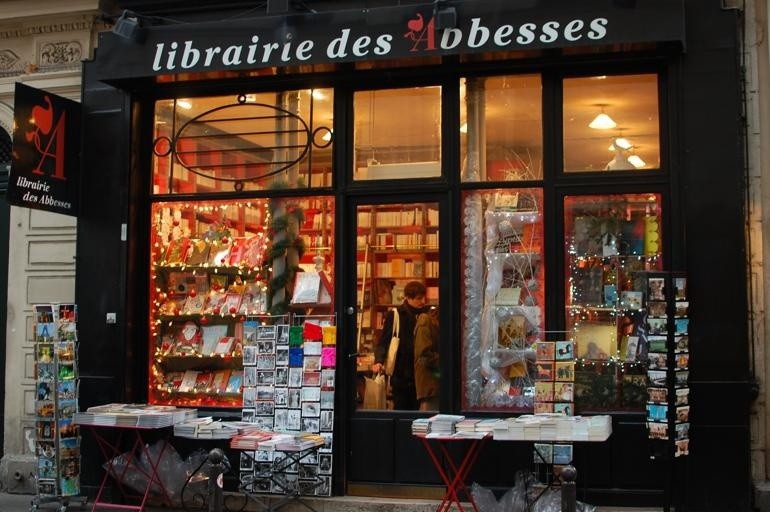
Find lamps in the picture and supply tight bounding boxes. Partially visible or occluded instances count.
[588,107,646,169]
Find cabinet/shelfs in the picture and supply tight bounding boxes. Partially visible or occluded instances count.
[156,122,440,406]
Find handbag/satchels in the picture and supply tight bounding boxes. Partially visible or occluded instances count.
[384,307,401,377]
[361,369,388,411]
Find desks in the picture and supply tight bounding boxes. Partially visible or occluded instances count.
[416,434,613,512]
[76,423,326,512]
